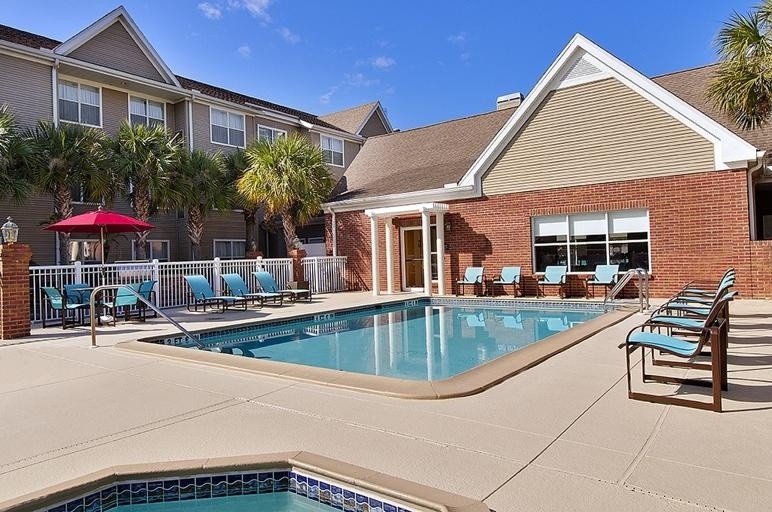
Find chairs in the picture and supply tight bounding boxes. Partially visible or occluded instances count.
[455,266,489,298]
[618,265,741,414]
[252,271,313,304]
[38,278,160,331]
[182,274,245,314]
[456,306,586,334]
[535,265,573,299]
[492,265,526,299]
[584,263,619,304]
[218,273,284,309]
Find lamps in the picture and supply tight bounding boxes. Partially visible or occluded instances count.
[1,215,20,244]
[292,235,302,250]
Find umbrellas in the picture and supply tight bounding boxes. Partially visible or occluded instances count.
[40,206,156,316]
